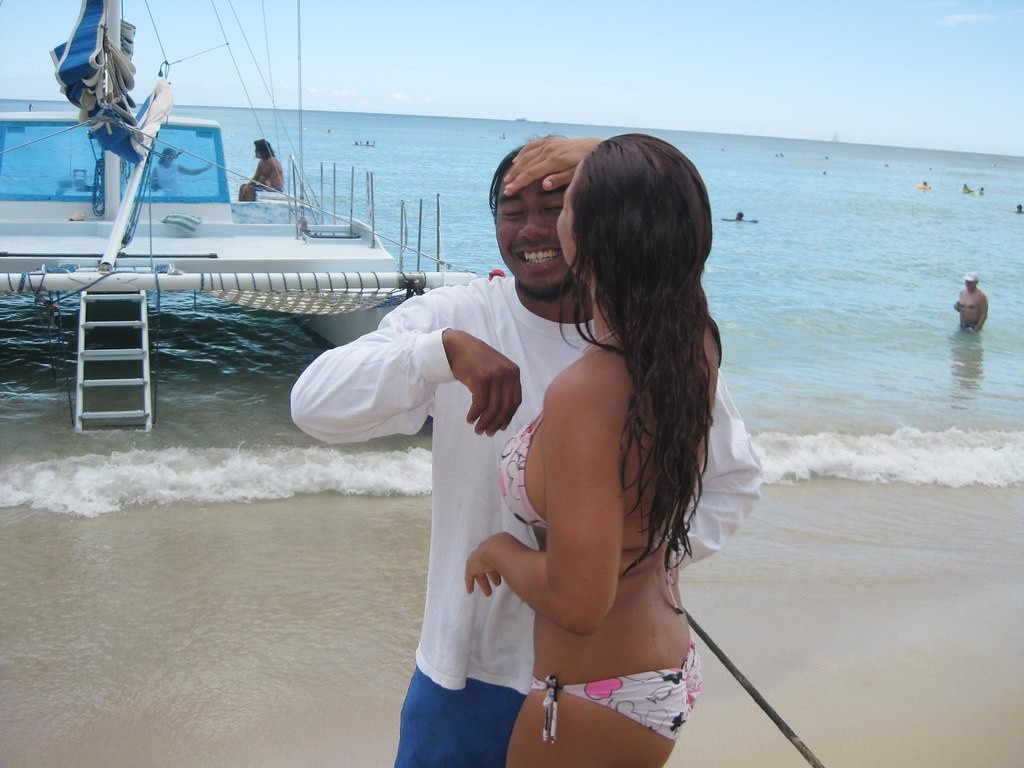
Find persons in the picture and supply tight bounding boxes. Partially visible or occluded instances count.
[960,184,972,193]
[1014,204,1024,213]
[238,137,284,203]
[952,273,989,333]
[922,181,928,187]
[722,211,759,224]
[978,187,984,196]
[290,133,765,768]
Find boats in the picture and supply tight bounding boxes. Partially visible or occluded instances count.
[1,0,505,419]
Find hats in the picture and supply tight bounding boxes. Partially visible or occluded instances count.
[964,271,979,281]
